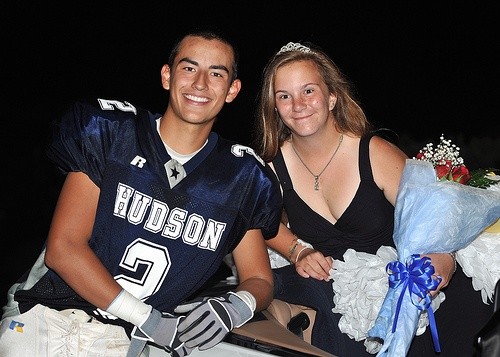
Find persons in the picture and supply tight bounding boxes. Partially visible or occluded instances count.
[0,26,284,357]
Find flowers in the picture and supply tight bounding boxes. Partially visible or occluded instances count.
[364,133,500,357]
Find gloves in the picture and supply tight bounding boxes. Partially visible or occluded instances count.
[173,292,254,351]
[127,309,195,357]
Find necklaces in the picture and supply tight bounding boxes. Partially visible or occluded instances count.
[449,252,458,274]
[289,135,345,192]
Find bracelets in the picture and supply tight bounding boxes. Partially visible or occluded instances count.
[250,40,500,357]
[287,237,312,264]
